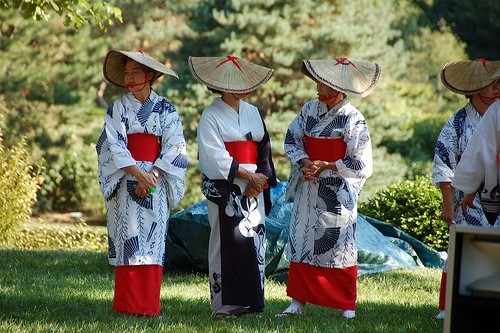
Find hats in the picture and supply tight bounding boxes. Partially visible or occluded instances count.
[301,56,381,99]
[188,54,275,94]
[442,58,500,97]
[102,49,179,88]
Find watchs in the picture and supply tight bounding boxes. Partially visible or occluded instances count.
[152,171,158,178]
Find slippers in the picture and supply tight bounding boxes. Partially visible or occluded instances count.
[275,311,306,319]
[341,316,357,322]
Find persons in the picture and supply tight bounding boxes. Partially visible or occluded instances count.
[432,61,500,318]
[189,56,278,317]
[284,57,380,318]
[451,100,500,214]
[97,49,187,317]
